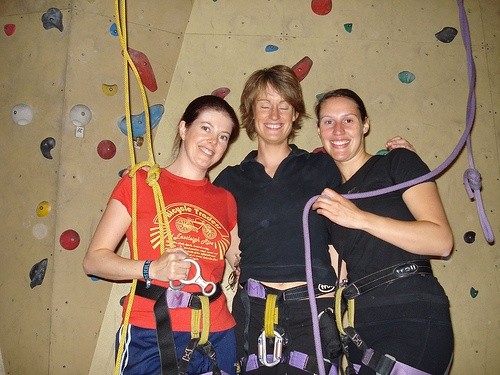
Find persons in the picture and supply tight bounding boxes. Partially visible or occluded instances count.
[82,95,243,375]
[313,86,456,375]
[119,63,414,375]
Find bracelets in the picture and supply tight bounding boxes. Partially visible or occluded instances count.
[142,259,155,289]
